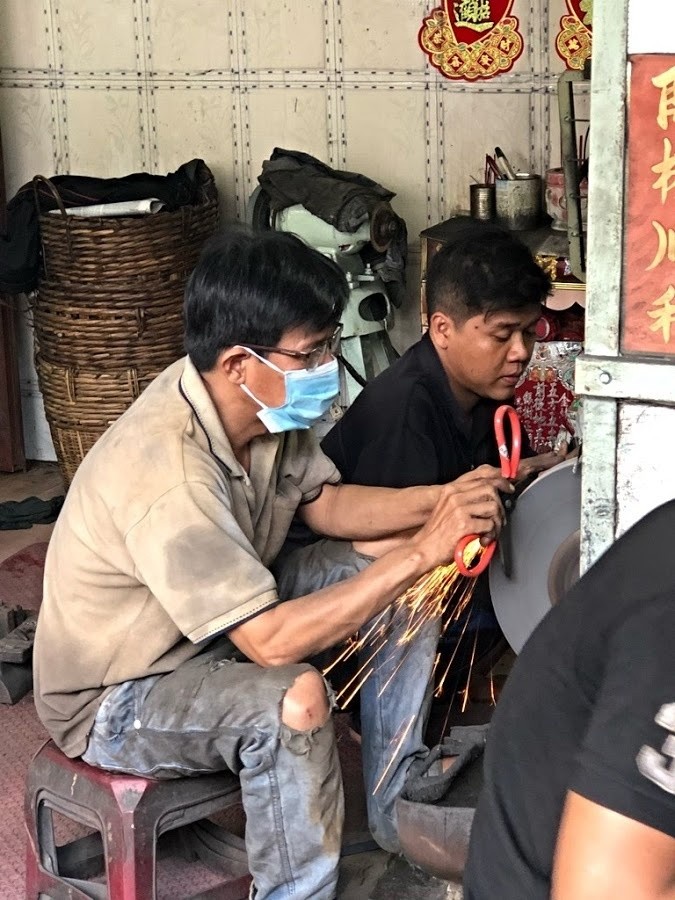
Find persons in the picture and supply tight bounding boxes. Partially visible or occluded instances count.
[30,225,517,900]
[320,217,582,736]
[462,496,675,899]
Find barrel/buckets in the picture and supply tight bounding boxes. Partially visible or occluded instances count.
[496,173,544,232]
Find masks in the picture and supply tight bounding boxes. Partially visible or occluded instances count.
[232,343,341,435]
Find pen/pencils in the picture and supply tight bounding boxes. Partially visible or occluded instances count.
[469,146,518,185]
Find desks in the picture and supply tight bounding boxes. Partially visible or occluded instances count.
[420,215,587,346]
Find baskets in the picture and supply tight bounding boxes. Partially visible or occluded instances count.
[23,170,226,499]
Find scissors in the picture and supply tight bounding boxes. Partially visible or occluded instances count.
[454,404,532,580]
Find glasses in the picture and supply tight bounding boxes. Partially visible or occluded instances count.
[230,322,345,374]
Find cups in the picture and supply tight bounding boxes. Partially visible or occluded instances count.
[543,165,587,233]
[469,184,494,221]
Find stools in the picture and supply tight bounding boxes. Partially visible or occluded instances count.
[24,737,252,900]
